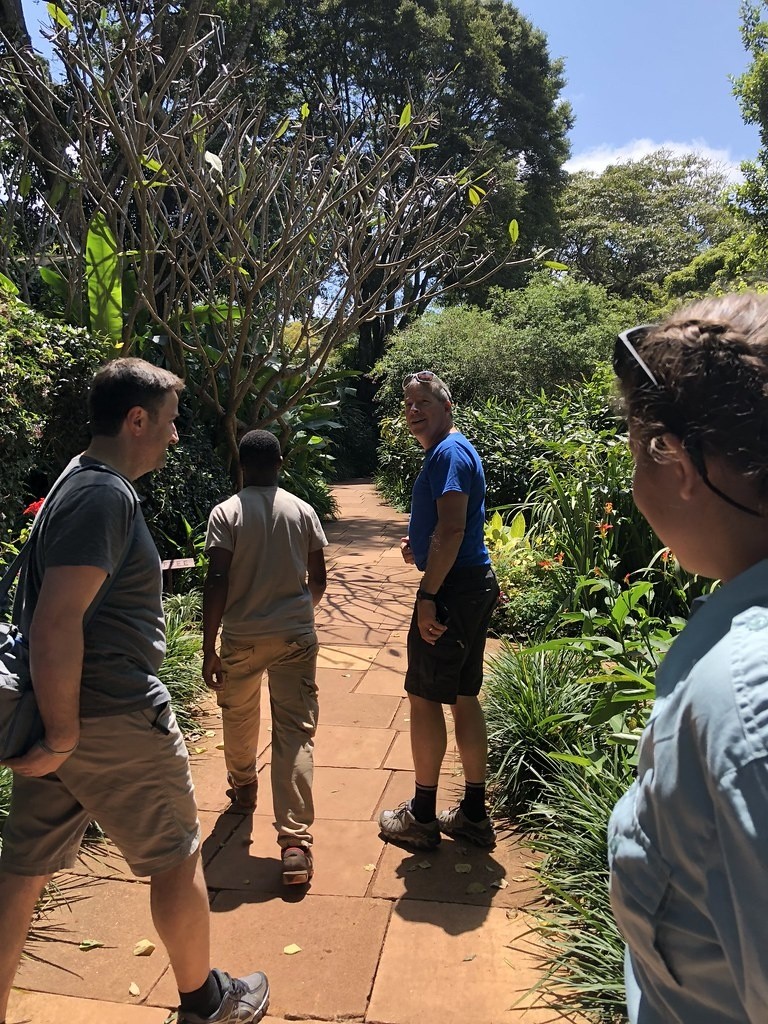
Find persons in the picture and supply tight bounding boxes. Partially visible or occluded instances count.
[604,298,768,1024]
[378,372,501,847]
[0,357,272,1024]
[201,431,330,883]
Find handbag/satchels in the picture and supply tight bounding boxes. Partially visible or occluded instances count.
[0,613,44,761]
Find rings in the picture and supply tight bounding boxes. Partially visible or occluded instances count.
[428,627,433,631]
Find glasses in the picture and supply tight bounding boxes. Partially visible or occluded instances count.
[612,324,660,389]
[402,370,452,403]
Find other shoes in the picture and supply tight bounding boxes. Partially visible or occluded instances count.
[234,790,257,813]
[280,846,314,884]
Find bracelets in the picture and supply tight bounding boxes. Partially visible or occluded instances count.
[37,738,80,755]
[415,589,435,601]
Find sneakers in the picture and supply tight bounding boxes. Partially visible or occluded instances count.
[175,967,270,1024]
[378,798,442,848]
[439,800,497,846]
